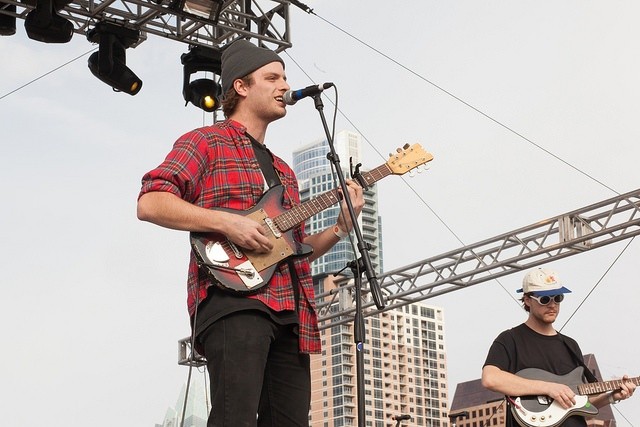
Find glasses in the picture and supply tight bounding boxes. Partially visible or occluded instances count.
[529,294,564,306]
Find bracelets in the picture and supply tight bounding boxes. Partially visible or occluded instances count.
[332,223,352,240]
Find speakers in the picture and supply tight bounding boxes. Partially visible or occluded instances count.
[449,354,616,427]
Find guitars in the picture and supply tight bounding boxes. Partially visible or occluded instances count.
[509,365,640,427]
[189,141,436,296]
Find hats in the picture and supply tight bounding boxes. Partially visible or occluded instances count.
[516,267,572,297]
[220,38,286,97]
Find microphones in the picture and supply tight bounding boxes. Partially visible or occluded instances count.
[197,261,255,277]
[283,82,333,104]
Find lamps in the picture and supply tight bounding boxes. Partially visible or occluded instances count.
[86,21,143,96]
[23,7,75,43]
[182,46,225,112]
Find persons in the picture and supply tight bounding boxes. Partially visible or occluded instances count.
[482,268,636,427]
[136,41,366,427]
